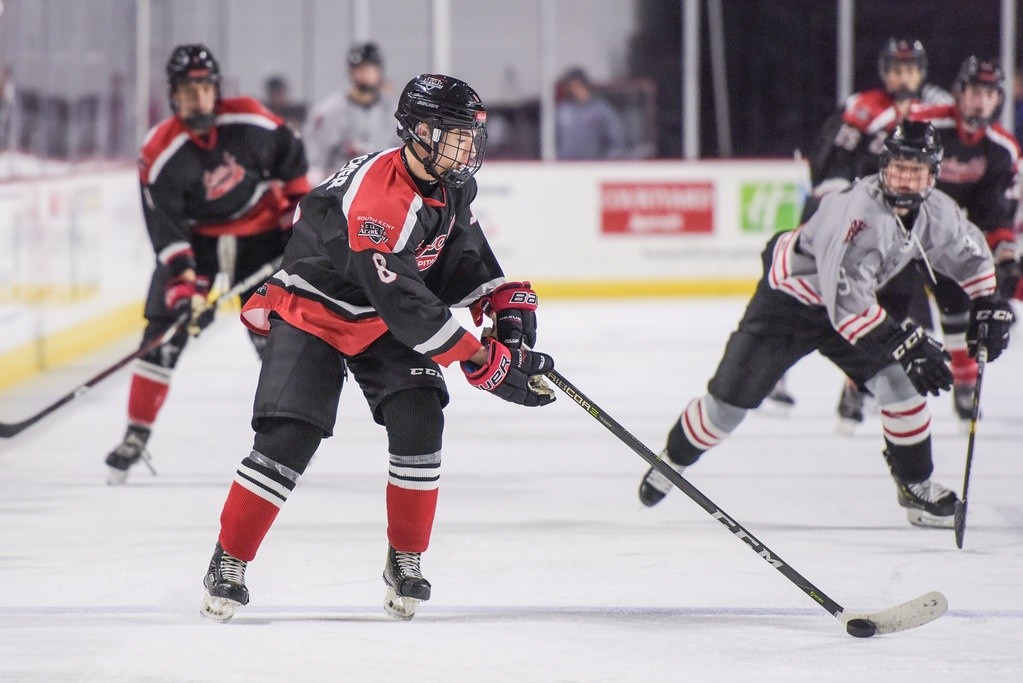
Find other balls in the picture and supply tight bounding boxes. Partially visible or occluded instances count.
[847,618,876,637]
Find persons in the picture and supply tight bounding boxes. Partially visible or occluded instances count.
[744,36,959,416]
[556,69,633,160]
[642,56,714,158]
[0,63,21,108]
[302,45,403,189]
[639,115,1013,528]
[104,45,313,487]
[198,70,559,624]
[836,50,1023,434]
[260,74,306,128]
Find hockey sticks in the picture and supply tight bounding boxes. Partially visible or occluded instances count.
[1,257,284,442]
[953,321,991,550]
[546,366,950,637]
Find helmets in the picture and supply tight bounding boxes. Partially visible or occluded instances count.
[951,52,1005,126]
[166,44,219,129]
[878,36,927,96]
[876,120,942,210]
[348,44,379,66]
[394,74,488,190]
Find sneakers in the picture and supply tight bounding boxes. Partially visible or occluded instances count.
[382,544,431,620]
[198,541,249,622]
[104,424,150,483]
[763,380,797,416]
[639,449,686,507]
[882,451,960,529]
[838,378,866,421]
[954,385,982,427]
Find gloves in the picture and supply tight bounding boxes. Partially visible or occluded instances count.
[468,281,537,350]
[459,336,556,407]
[965,299,1014,362]
[883,317,955,397]
[165,277,216,338]
